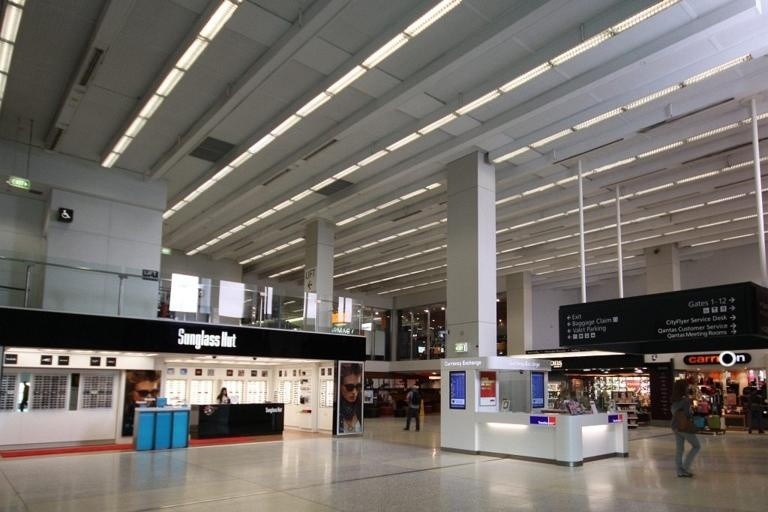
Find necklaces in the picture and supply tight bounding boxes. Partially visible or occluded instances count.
[346,418,354,431]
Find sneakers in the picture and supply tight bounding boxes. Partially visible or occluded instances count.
[676,471,694,478]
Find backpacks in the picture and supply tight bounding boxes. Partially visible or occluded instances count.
[411,390,421,406]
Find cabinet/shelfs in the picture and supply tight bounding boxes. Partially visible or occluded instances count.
[616,402,639,427]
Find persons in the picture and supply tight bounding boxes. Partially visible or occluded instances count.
[670,379,701,478]
[747,391,765,434]
[123,370,160,437]
[340,364,362,434]
[372,383,440,410]
[217,388,230,404]
[402,384,423,431]
